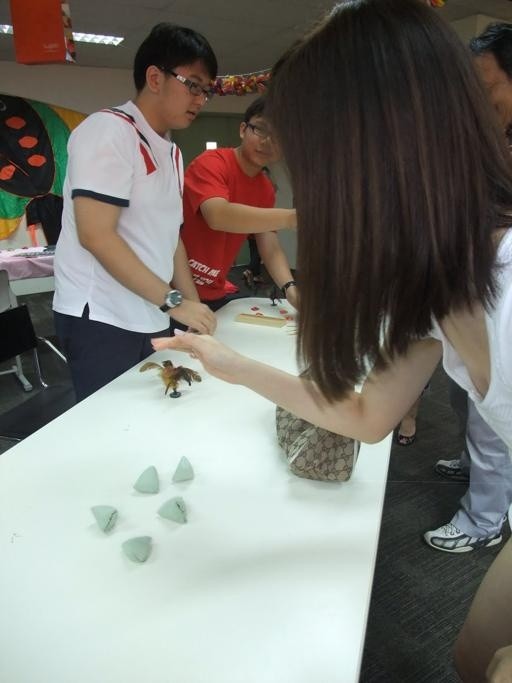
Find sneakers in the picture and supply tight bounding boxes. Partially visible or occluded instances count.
[435,460,469,480]
[424,522,503,553]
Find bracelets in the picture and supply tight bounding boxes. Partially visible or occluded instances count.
[282,280,296,298]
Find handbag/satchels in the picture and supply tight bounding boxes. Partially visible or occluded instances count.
[276,367,360,482]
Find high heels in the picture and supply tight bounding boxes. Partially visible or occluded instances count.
[242,270,266,288]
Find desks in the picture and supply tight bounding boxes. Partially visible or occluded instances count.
[7,275,68,392]
[1,297,397,683]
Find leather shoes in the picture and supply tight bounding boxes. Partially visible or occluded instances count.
[398,431,417,445]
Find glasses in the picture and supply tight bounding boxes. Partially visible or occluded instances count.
[159,66,215,100]
[246,122,278,144]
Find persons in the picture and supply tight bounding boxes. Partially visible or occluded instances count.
[420,19,511,554]
[46,19,218,404]
[396,397,420,445]
[177,94,302,314]
[148,2,510,682]
[241,233,272,289]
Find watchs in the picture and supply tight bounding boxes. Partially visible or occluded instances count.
[158,287,183,313]
